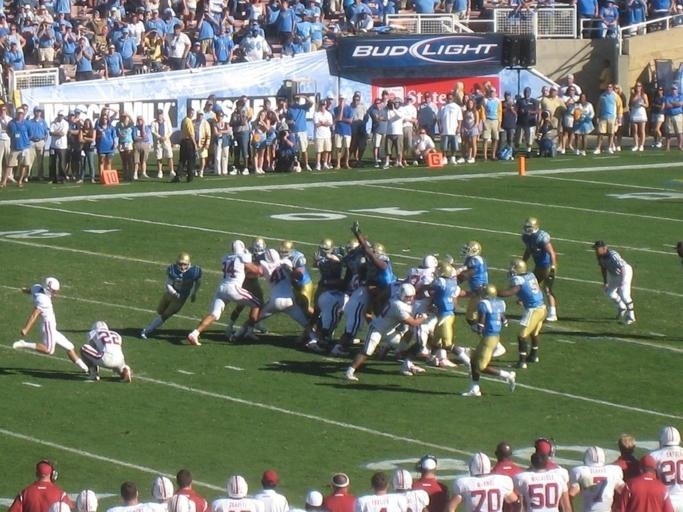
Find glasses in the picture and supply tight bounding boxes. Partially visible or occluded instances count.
[0,83,683,127]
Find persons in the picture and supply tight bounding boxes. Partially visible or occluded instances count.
[497,259,547,368]
[190,223,517,397]
[673,240,683,265]
[80,321,131,383]
[590,240,636,325]
[141,252,202,340]
[0,1,683,189]
[304,454,450,512]
[1,277,89,374]
[9,458,290,512]
[517,217,557,321]
[448,425,683,512]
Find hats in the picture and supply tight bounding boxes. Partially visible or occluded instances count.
[593,240,605,247]
[0,0,625,80]
[37,463,52,476]
[263,470,281,486]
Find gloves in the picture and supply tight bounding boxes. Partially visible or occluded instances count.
[547,269,555,283]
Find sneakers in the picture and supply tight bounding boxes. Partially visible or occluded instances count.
[12,340,23,350]
[84,370,89,374]
[87,375,100,381]
[616,309,635,326]
[137,302,559,397]
[120,367,129,381]
[0,127,679,189]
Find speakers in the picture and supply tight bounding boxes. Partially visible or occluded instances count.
[501,34,519,67]
[519,33,537,67]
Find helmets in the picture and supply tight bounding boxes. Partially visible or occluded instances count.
[471,426,681,477]
[77,490,98,511]
[305,457,438,504]
[228,475,248,498]
[49,501,69,510]
[44,278,59,289]
[175,238,528,300]
[153,477,173,502]
[521,218,538,235]
